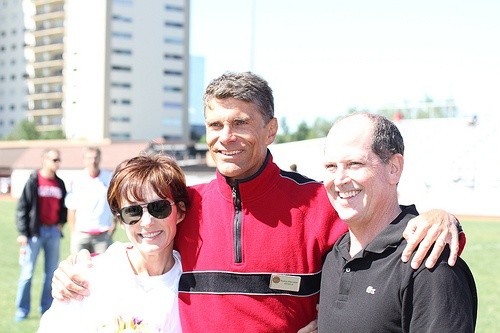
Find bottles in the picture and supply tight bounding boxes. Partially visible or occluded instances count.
[18,243,27,266]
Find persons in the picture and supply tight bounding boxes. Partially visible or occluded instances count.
[15,147,67,320]
[52,72,466,333]
[37,154,319,333]
[64,147,118,255]
[318,113,478,333]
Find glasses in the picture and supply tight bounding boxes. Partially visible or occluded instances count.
[49,159,61,162]
[116,200,177,225]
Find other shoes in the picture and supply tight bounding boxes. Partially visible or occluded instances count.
[15,309,27,322]
